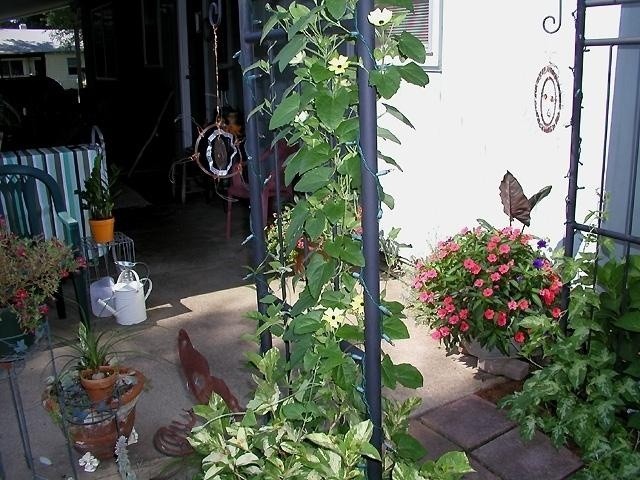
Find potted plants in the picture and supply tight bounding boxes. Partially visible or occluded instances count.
[74,148,123,242]
[41,365,144,459]
[40,292,183,403]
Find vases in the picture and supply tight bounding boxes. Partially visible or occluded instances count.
[0,306,35,362]
[459,333,529,358]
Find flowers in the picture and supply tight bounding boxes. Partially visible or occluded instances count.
[403,223,561,355]
[0,216,87,337]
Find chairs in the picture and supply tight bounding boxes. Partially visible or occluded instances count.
[226,139,287,240]
